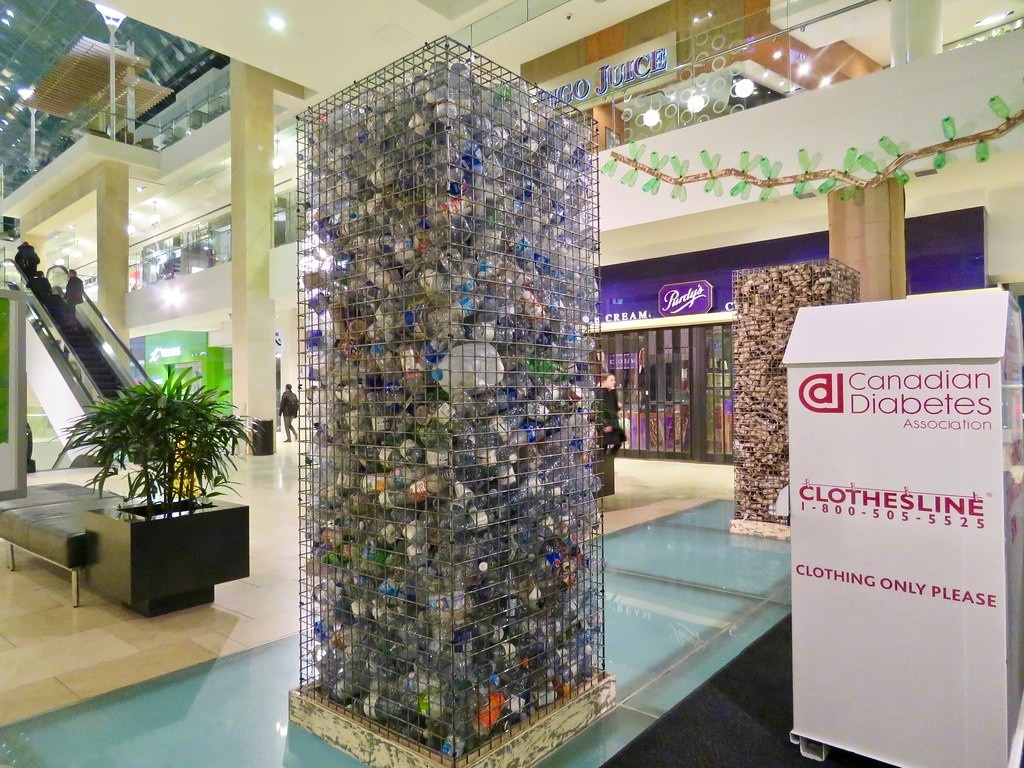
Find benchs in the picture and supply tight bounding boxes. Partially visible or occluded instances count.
[0,482,133,609]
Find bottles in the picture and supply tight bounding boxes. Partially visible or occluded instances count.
[299,35,606,755]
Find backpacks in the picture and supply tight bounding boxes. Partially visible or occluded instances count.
[285,392,298,415]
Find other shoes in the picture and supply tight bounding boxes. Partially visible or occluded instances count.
[295,434,298,441]
[284,439,291,442]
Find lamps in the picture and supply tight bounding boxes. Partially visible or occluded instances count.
[70,200,161,260]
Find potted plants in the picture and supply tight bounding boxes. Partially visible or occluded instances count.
[48,361,265,620]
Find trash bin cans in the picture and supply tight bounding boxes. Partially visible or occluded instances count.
[251,419,274,456]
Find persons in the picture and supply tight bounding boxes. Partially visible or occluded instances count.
[596,373,627,456]
[63,269,83,329]
[279,384,298,442]
[15,241,40,276]
[25,271,53,307]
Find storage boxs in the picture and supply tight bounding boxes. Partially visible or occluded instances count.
[287,34,618,768]
[729,256,863,545]
